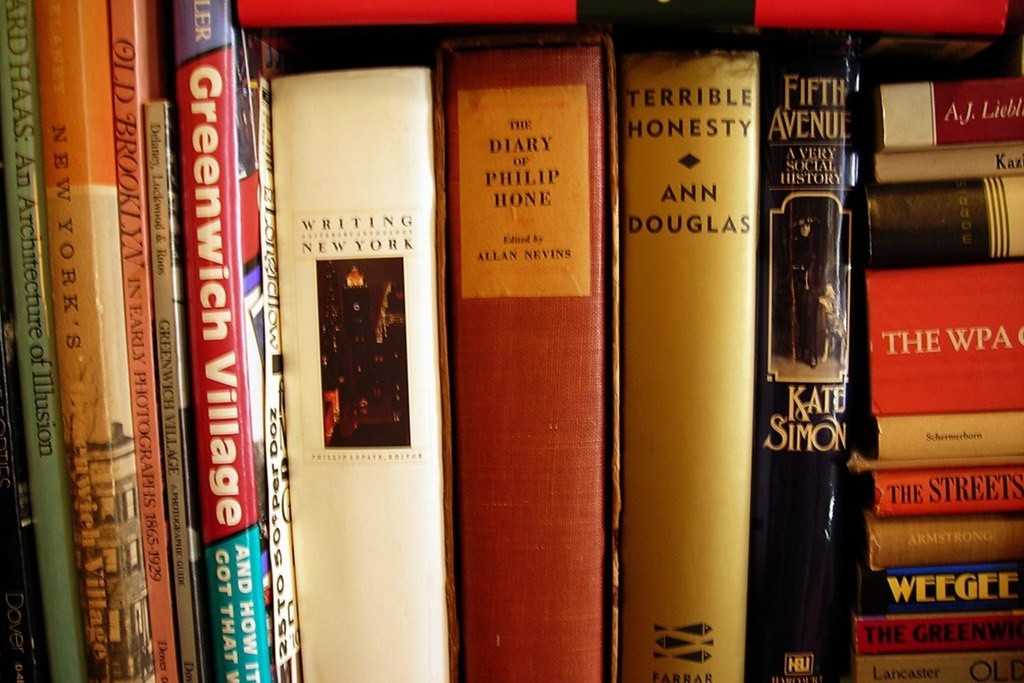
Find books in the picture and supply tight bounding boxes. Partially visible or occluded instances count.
[0,0,1024,683]
